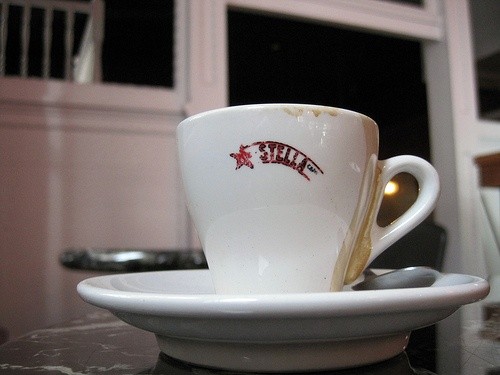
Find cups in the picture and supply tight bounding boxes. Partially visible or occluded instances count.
[176,104,442,297]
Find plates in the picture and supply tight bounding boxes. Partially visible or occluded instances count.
[76,267,489,372]
